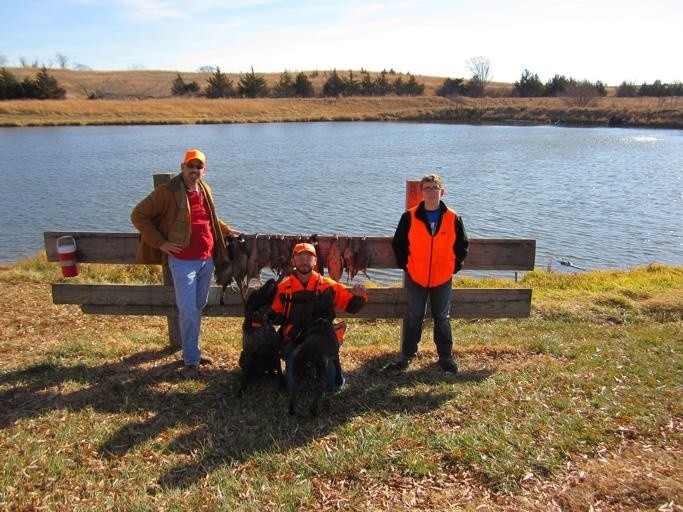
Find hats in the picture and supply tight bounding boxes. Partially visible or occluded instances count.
[292,243,316,257]
[185,150,206,165]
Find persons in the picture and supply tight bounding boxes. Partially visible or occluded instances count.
[391,175,468,374]
[130,150,240,368]
[268,243,369,395]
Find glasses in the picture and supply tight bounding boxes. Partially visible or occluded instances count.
[186,163,204,170]
[422,184,442,194]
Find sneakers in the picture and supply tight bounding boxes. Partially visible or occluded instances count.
[182,353,214,363]
[439,356,457,374]
[400,357,415,369]
[183,365,199,380]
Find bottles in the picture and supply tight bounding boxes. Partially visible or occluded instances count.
[56,235,80,278]
